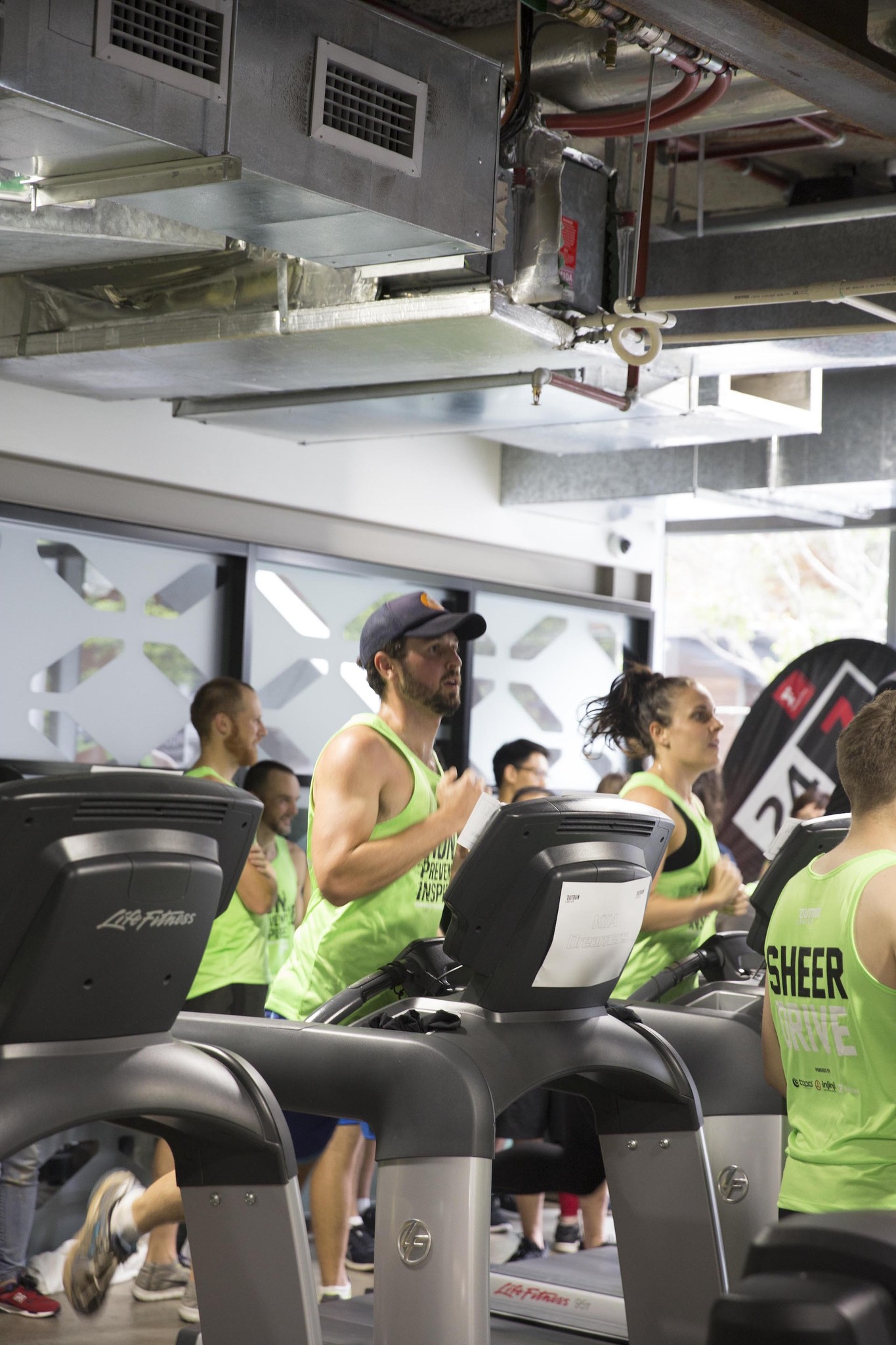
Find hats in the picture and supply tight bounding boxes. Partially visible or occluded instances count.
[359,593,487,669]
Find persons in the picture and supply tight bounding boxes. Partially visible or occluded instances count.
[131,676,279,1301]
[0,668,896,1322]
[578,661,750,1008]
[62,589,487,1319]
[762,684,895,1226]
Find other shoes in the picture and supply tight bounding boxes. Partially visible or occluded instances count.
[507,1242,548,1263]
[490,1199,512,1233]
[554,1220,580,1252]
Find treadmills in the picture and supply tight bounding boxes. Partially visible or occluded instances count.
[170,795,729,1345]
[0,771,324,1345]
[490,812,853,1344]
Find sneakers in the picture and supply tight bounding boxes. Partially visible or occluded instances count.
[133,1258,191,1302]
[178,1275,200,1323]
[361,1206,375,1233]
[62,1167,140,1315]
[0,1284,60,1318]
[343,1225,375,1272]
